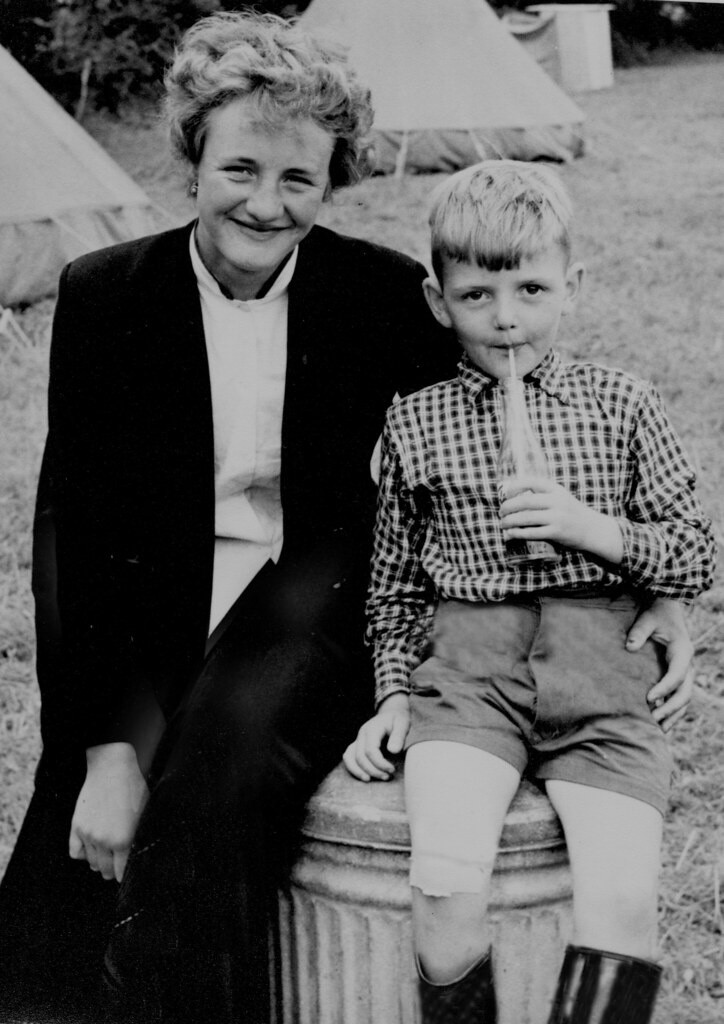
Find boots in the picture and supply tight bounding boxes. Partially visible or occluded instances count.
[414,943,497,1024]
[547,944,664,1024]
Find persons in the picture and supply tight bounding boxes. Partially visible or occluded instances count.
[344,157,716,1024]
[0,13,695,1024]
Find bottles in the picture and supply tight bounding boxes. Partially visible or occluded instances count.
[497,377,562,570]
